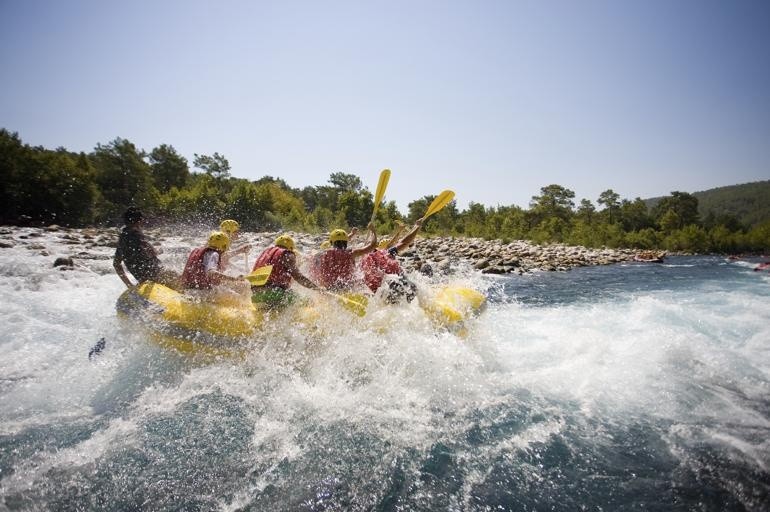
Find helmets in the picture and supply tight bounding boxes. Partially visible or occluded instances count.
[220,220,240,234]
[207,231,230,251]
[319,228,349,249]
[275,236,294,250]
[379,239,391,249]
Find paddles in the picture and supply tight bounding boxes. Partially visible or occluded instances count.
[324,291,368,316]
[399,191,455,243]
[244,265,273,285]
[364,169,391,246]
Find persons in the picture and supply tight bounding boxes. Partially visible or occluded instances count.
[112,206,181,297]
[302,222,421,310]
[218,220,253,277]
[251,235,329,312]
[178,227,252,308]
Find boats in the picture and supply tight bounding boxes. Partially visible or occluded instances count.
[114,278,490,359]
